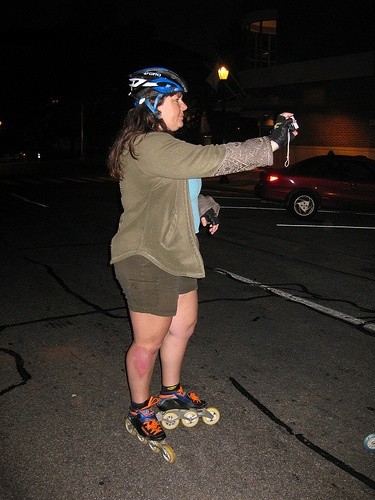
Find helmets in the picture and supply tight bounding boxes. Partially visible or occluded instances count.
[128,67,187,107]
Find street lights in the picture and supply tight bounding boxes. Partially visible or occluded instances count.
[218,64,231,184]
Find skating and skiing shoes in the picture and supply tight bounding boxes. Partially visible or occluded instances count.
[157,385,220,430]
[125,400,176,464]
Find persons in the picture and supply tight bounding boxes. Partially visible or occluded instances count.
[110,67,298,465]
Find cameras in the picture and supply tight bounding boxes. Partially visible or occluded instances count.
[288,115,299,132]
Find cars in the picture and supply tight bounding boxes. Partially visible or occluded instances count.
[12,148,44,161]
[252,150,375,221]
